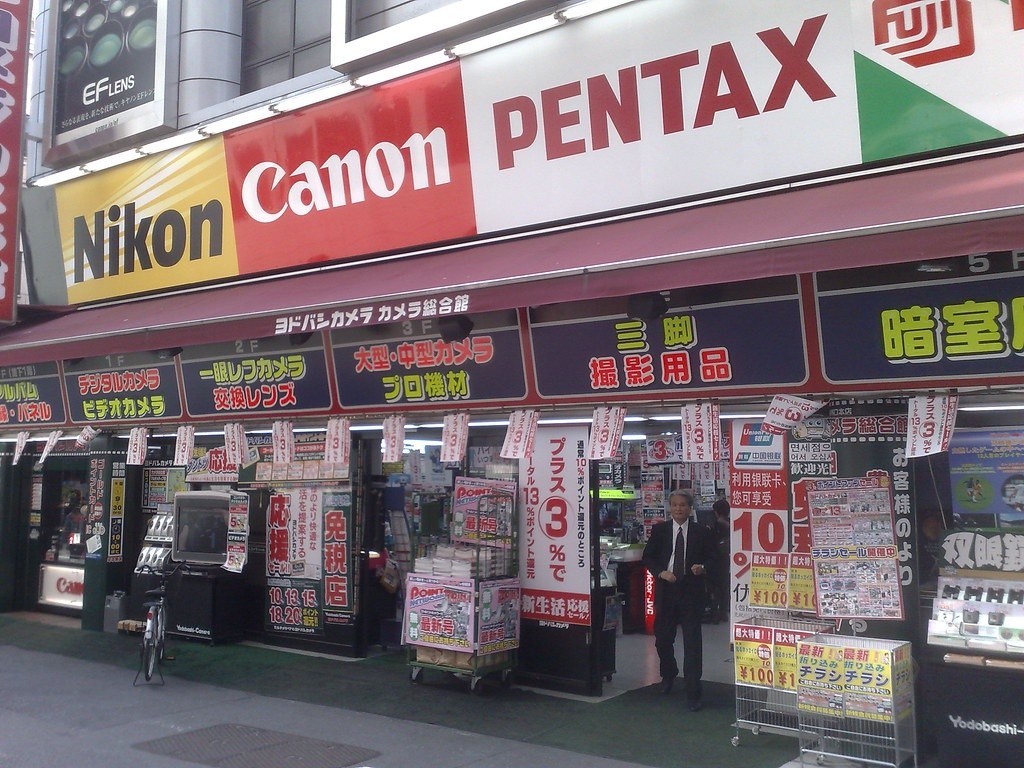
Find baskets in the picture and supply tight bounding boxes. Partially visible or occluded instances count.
[138,564,181,598]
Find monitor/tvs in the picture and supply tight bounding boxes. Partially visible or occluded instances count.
[172,491,248,565]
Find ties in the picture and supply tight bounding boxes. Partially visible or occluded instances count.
[673,527,684,585]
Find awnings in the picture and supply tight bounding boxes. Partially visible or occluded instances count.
[0,148,1024,366]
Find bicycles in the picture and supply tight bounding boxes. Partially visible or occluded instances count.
[132,560,193,688]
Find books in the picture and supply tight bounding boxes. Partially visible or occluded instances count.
[414,544,512,582]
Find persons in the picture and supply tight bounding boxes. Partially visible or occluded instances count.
[644,489,720,711]
[185,514,227,553]
[704,499,729,623]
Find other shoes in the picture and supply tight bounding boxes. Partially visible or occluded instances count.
[721,611,729,621]
[684,677,699,704]
[710,612,720,622]
[660,668,679,695]
